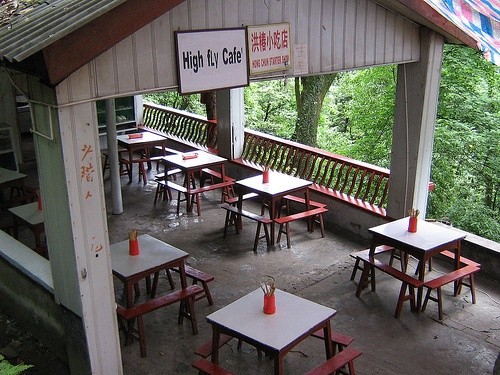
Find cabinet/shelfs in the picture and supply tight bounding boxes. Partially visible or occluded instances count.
[96,95,138,136]
[0,123,19,172]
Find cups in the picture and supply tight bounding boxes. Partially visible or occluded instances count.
[264,293,276,314]
[408,217,417,232]
[129,240,139,256]
[263,171,269,182]
[38,197,42,210]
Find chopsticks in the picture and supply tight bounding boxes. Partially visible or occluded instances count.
[262,166,269,171]
[408,209,420,217]
[128,229,136,240]
[261,282,275,296]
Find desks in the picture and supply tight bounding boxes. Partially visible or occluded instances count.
[9,201,45,254]
[234,172,313,246]
[117,131,168,182]
[205,285,337,375]
[0,167,27,207]
[110,233,189,340]
[368,214,467,312]
[161,150,226,212]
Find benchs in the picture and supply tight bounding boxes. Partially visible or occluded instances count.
[0,103,481,375]
